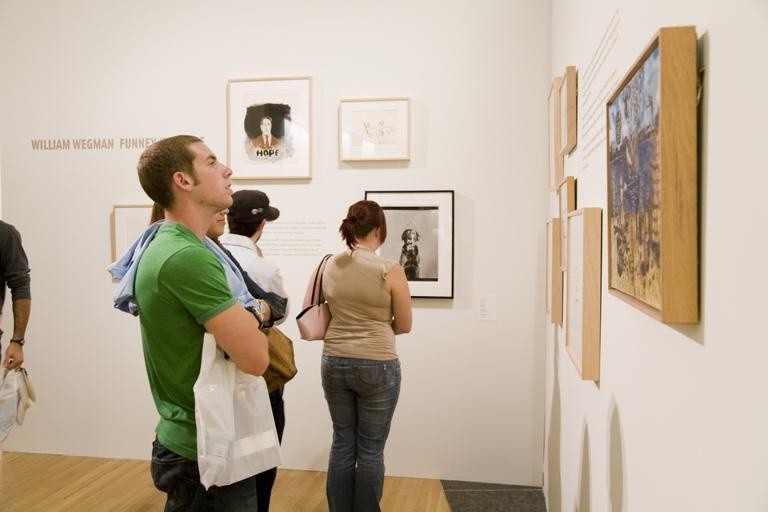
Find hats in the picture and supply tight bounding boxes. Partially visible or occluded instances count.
[227,190,279,224]
[16,367,36,426]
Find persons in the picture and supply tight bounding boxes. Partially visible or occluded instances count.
[251,115,280,149]
[218,189,289,327]
[0,219,32,370]
[399,228,421,279]
[133,134,270,512]
[319,199,414,512]
[149,200,288,512]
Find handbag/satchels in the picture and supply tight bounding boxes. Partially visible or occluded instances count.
[296,254,333,342]
[192,331,282,492]
[258,326,297,394]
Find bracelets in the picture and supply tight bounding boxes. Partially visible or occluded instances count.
[10,338,25,346]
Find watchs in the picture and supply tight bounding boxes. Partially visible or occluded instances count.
[256,299,266,313]
[244,305,264,329]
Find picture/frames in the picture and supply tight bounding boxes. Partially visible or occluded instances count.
[223,75,315,182]
[546,64,603,383]
[363,188,456,301]
[111,202,159,284]
[337,97,412,165]
[603,25,698,329]
